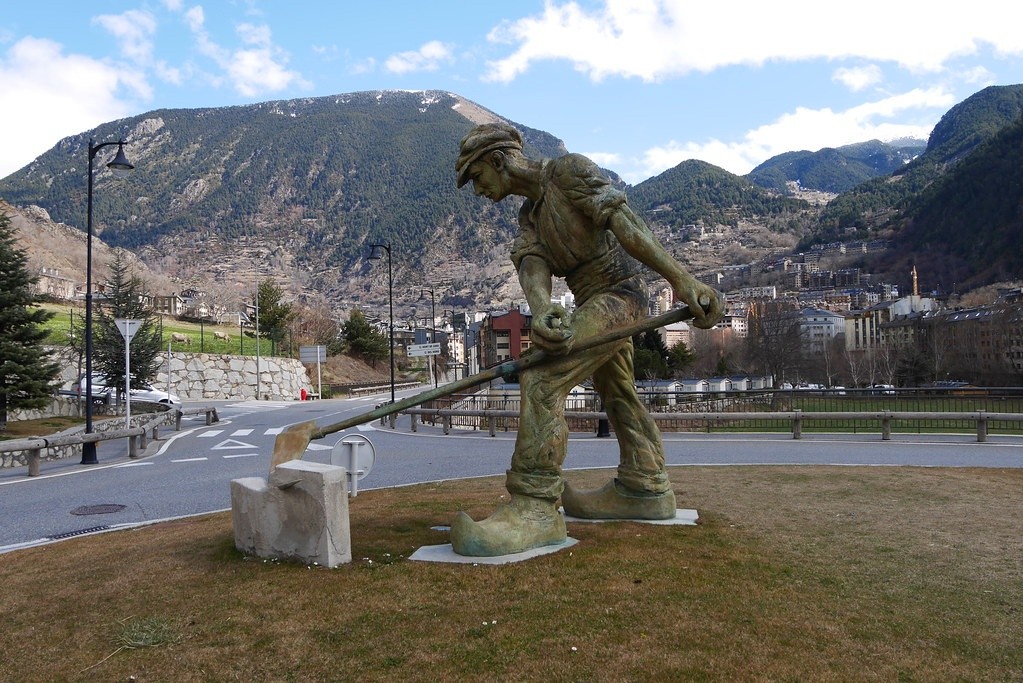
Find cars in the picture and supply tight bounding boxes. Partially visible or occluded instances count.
[780,382,894,395]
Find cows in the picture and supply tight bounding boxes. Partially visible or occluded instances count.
[214,332,228,340]
[172,333,192,345]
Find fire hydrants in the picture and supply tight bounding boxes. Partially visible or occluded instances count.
[301,389,307,401]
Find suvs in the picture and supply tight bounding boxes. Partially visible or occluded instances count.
[72,375,182,411]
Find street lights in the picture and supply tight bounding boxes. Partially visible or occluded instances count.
[465,329,476,376]
[246,305,261,399]
[80,140,136,464]
[443,309,458,382]
[419,289,437,389]
[367,244,394,403]
